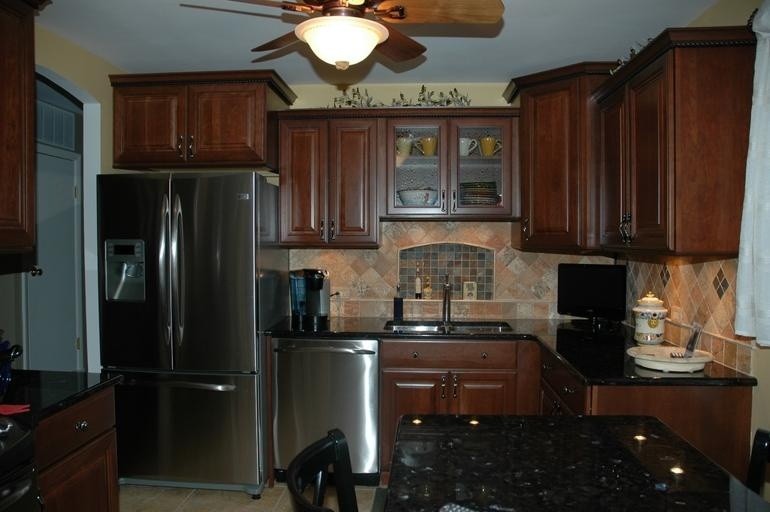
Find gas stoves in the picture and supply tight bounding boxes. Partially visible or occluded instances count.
[0,413,35,487]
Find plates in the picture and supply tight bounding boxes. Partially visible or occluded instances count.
[633,366,705,381]
[459,182,499,207]
[628,345,713,372]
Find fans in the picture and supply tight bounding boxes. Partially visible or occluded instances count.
[229,0,506,61]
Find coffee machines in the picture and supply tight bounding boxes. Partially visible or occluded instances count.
[289,269,333,325]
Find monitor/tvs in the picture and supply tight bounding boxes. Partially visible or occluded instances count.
[558,262,627,334]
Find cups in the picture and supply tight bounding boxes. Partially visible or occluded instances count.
[415,136,438,157]
[396,137,413,157]
[480,138,502,155]
[459,137,477,156]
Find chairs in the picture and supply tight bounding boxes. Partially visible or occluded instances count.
[286,428,358,512]
[746,428,770,496]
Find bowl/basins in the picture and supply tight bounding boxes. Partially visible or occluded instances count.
[397,189,438,206]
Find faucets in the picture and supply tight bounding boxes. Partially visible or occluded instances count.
[441,282,452,323]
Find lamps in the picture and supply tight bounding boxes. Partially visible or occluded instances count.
[294,0,390,70]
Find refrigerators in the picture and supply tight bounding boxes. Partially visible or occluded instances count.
[94,170,280,497]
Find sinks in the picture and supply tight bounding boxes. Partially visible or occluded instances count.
[453,325,503,335]
[391,325,439,332]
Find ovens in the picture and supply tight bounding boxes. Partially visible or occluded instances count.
[271,338,379,483]
[1,467,41,510]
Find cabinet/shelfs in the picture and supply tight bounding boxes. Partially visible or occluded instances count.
[540,346,752,485]
[35,388,119,512]
[271,107,521,248]
[0,0,54,274]
[378,339,540,484]
[107,68,299,173]
[503,60,624,254]
[592,27,757,265]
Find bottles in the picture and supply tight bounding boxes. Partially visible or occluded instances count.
[632,291,668,346]
[424,275,433,299]
[414,261,422,299]
